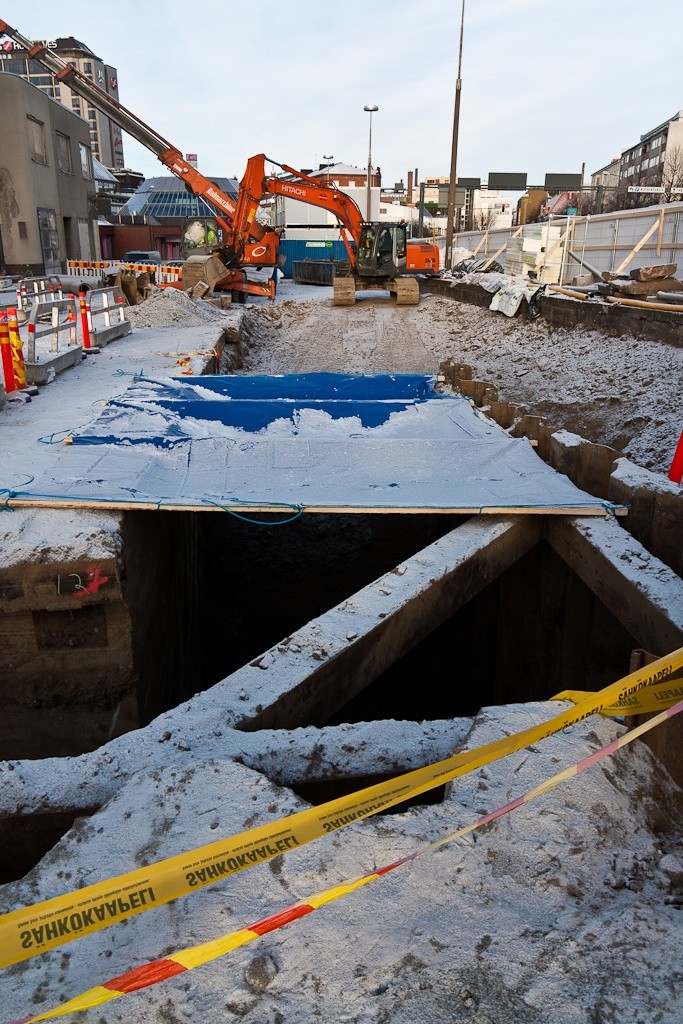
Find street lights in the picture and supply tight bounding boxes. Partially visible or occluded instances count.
[363,104,380,222]
[602,170,633,209]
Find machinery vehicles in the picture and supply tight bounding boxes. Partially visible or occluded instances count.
[0,18,282,305]
[180,152,440,307]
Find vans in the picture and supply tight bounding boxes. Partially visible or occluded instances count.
[122,250,161,266]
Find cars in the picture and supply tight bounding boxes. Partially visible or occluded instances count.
[161,259,185,268]
[134,259,157,266]
